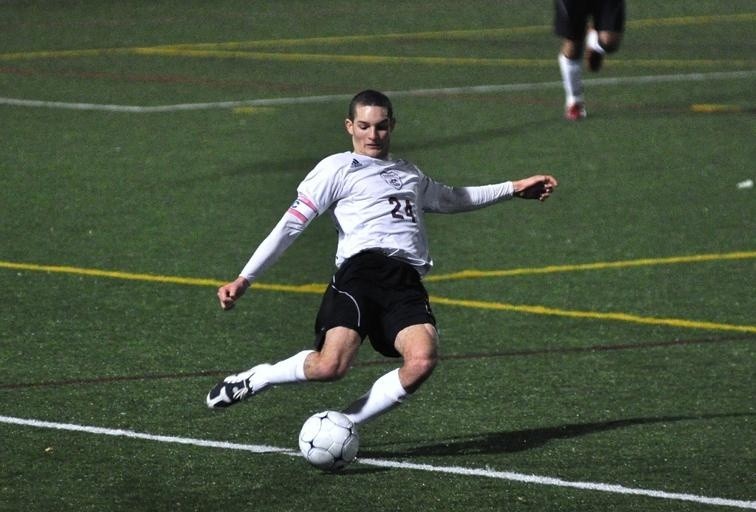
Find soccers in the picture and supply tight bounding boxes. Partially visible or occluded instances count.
[298,410,360,471]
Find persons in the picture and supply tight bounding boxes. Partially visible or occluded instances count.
[553,0,626,121]
[204,89,559,430]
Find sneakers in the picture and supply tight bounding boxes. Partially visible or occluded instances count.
[206,363,272,409]
[565,95,587,120]
[584,23,601,72]
